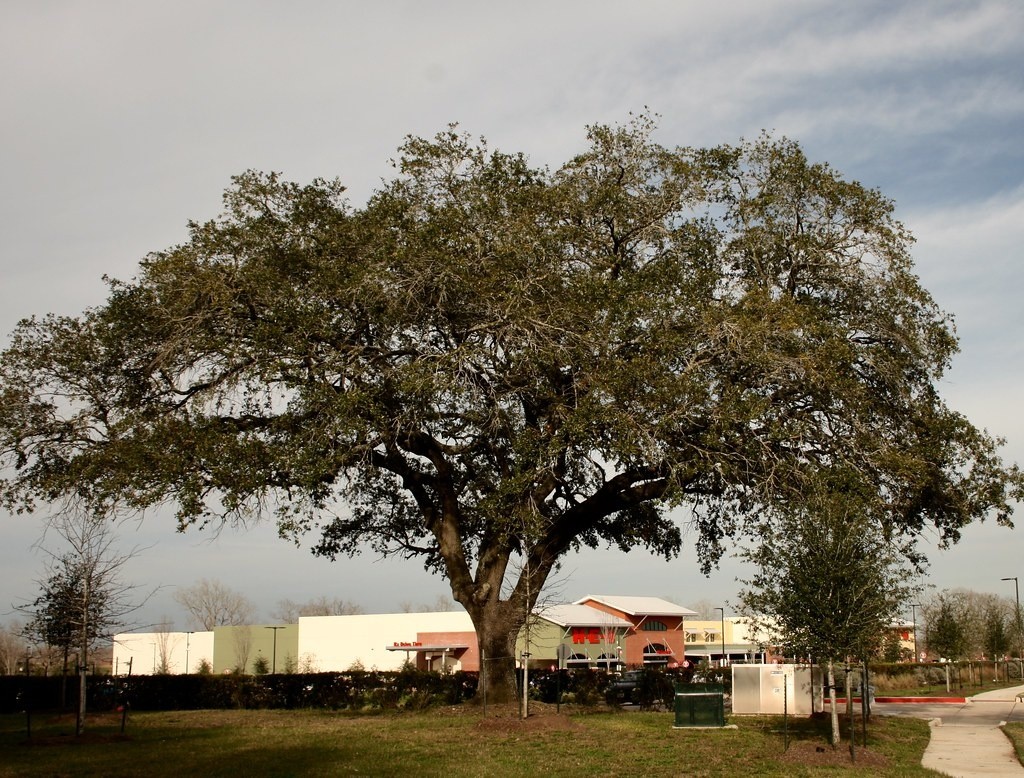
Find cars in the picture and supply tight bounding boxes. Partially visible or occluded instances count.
[605,669,675,706]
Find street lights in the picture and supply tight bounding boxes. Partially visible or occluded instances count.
[909,604,924,662]
[184,631,194,673]
[264,626,285,674]
[1002,577,1023,677]
[714,607,726,693]
[149,643,156,673]
